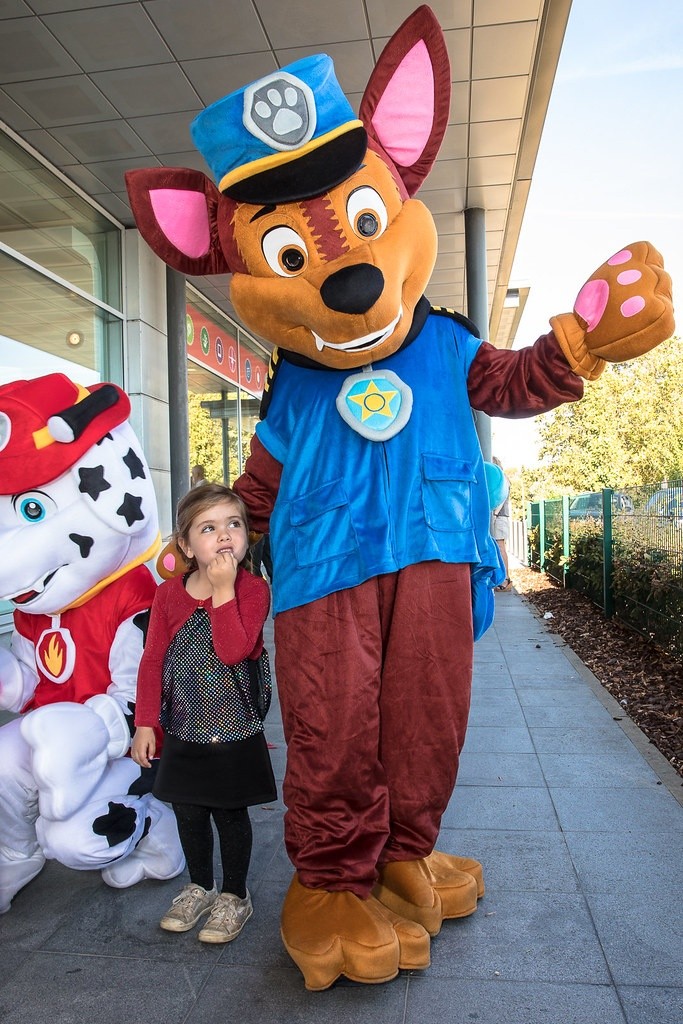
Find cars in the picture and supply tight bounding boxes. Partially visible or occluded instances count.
[644,486,683,519]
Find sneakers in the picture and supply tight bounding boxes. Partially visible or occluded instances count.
[198,888,254,942]
[160,879,217,932]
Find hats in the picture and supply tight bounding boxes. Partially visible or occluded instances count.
[0,373,131,494]
[190,51,370,204]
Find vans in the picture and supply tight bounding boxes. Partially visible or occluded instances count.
[567,492,634,518]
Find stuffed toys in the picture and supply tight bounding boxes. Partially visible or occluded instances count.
[0,373,184,916]
[125,2,676,991]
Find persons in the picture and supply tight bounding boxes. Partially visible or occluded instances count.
[129,483,278,943]
[490,454,513,593]
[191,464,210,488]
